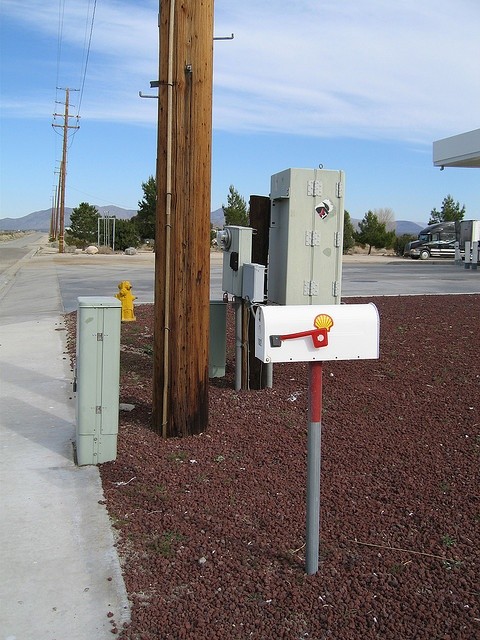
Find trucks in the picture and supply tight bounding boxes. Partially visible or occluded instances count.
[395,221,478,260]
[455,224,479,252]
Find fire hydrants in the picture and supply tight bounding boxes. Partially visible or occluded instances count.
[114,281,138,323]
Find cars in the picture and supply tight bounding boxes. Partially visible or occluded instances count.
[406,237,469,262]
